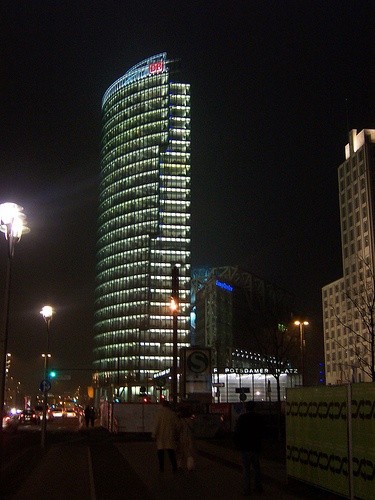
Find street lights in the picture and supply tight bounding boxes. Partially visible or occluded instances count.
[0,200,32,431]
[40,305,56,449]
[294,320,310,374]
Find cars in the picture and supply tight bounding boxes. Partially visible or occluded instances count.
[2,407,78,433]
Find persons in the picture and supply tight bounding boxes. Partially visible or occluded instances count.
[233,400,267,495]
[84,405,97,429]
[151,400,198,475]
